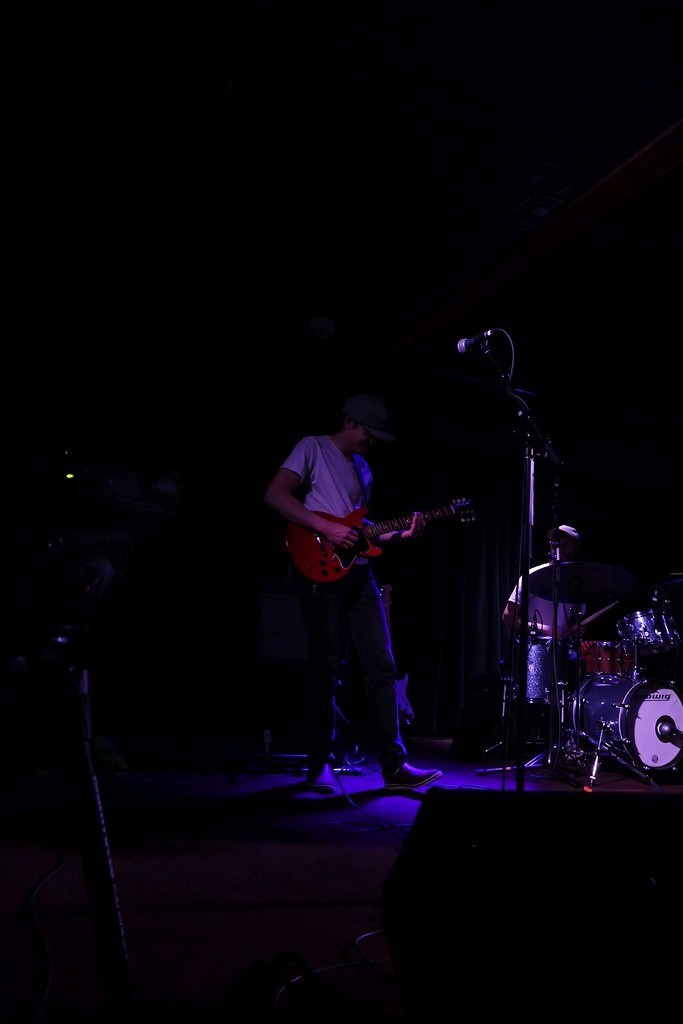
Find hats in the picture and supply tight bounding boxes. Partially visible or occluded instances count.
[548,525,581,546]
[344,392,397,442]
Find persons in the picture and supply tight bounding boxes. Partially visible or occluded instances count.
[264,396,443,795]
[500,523,635,644]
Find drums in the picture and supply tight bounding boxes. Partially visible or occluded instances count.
[567,675,683,771]
[615,607,682,657]
[512,636,553,705]
[579,640,635,675]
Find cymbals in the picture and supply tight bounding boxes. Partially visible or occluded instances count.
[549,560,578,567]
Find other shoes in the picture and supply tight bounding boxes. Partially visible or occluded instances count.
[306,754,336,791]
[385,762,443,787]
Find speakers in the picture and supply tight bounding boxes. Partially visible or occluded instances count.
[381,782,682,1020]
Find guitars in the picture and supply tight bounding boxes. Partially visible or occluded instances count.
[284,496,478,584]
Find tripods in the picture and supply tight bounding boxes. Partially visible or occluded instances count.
[476,456,584,790]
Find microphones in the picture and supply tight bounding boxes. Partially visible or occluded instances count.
[456,329,493,353]
